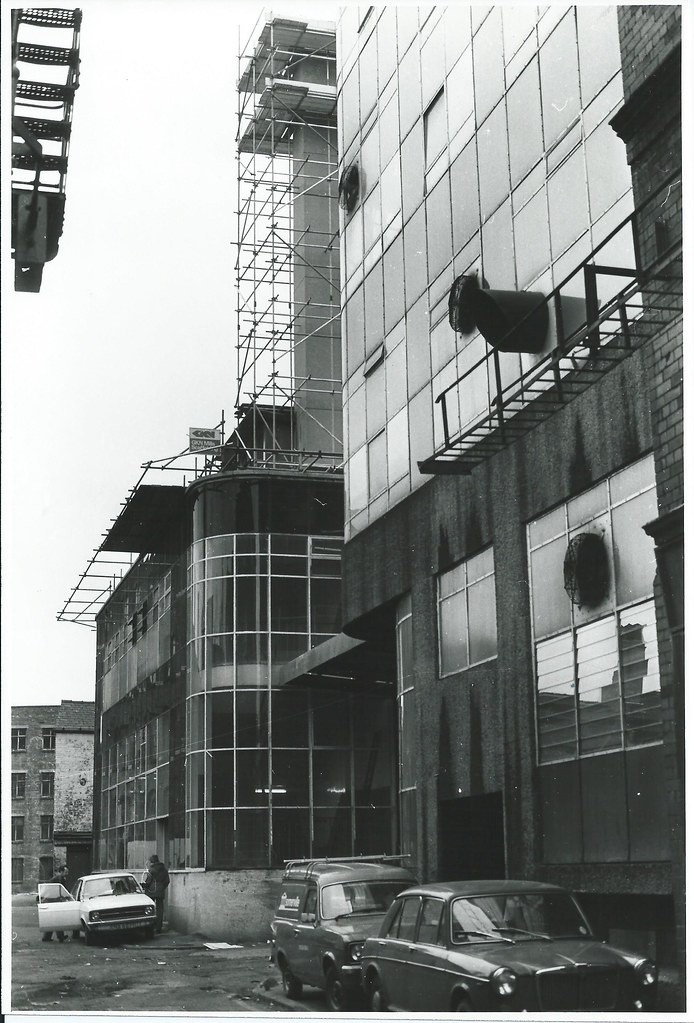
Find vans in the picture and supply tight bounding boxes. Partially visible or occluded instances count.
[272,856,422,1012]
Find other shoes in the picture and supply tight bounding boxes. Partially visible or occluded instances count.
[60,935,67,942]
[42,938,53,941]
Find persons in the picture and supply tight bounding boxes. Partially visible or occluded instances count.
[140,855,170,934]
[36,865,69,942]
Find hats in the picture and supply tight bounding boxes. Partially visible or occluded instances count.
[149,854,159,863]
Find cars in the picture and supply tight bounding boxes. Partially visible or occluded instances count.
[38,872,156,945]
[361,881,658,1012]
